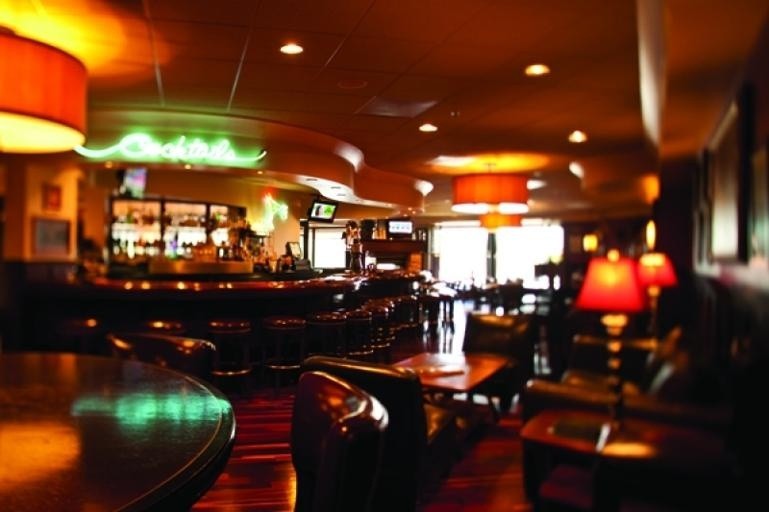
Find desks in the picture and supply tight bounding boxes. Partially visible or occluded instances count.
[0,351,237,512]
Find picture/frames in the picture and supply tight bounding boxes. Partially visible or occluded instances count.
[32,216,71,257]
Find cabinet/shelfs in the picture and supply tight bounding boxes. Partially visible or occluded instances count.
[106,195,248,270]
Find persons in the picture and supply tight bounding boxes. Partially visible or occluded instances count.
[312,204,327,219]
[545,269,587,377]
[77,237,105,264]
[609,312,714,406]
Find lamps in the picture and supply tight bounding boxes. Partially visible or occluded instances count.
[638,253,676,312]
[0,19,87,155]
[451,161,531,213]
[574,258,641,401]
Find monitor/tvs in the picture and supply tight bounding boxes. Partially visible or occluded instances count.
[308,199,338,224]
[285,242,301,258]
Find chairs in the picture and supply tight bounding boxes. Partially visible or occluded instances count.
[289,371,391,511]
[520,408,670,509]
[106,283,768,387]
[301,355,467,512]
